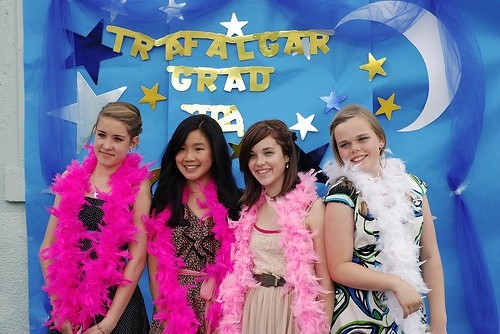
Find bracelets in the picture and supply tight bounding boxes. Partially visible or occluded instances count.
[95,322,107,334]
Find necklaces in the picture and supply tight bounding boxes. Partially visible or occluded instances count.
[89,166,115,198]
[263,191,284,201]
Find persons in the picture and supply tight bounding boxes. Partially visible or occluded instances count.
[233,118,336,334]
[323,104,447,334]
[38,102,154,334]
[145,113,246,334]
